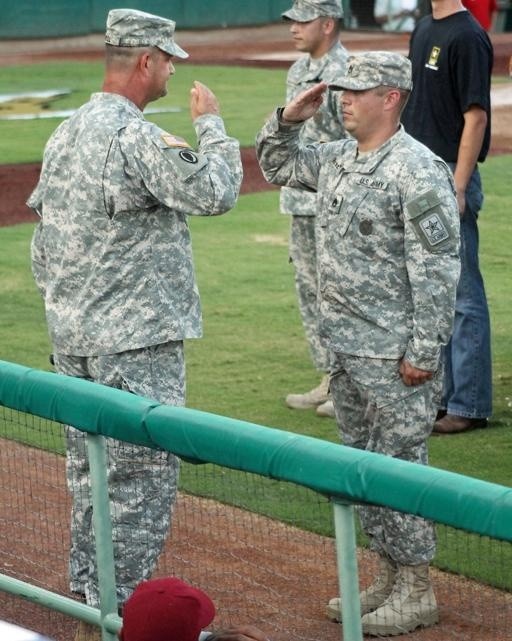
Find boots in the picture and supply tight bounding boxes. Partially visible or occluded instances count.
[285,371,338,420]
[326,553,441,637]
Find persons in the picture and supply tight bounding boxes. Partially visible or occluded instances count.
[256,52,463,637]
[279,0,358,419]
[25,7,245,641]
[399,0,495,433]
[205,625,269,641]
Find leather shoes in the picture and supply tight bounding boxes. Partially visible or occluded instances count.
[433,414,488,435]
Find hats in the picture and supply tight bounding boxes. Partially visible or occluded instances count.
[328,50,415,94]
[103,8,190,60]
[281,0,346,25]
